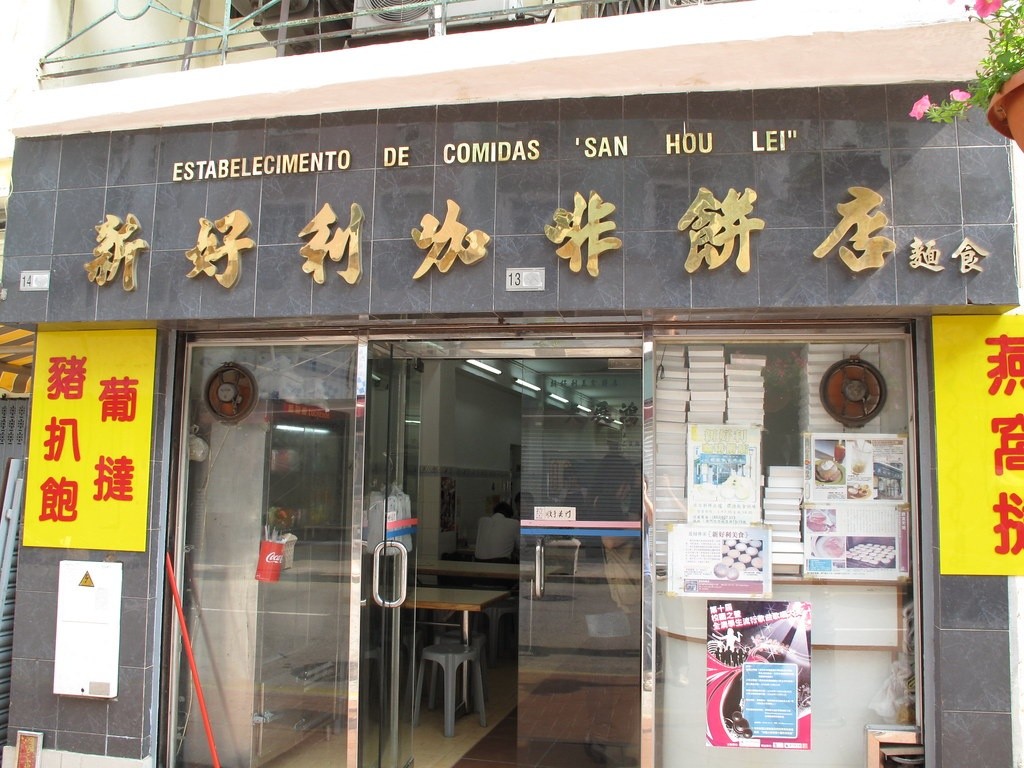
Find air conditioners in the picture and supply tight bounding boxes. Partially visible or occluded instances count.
[351,0,520,38]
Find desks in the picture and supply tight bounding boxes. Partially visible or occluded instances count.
[370,559,562,713]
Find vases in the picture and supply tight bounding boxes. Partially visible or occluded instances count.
[986,68,1024,152]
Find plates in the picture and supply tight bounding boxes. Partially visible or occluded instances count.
[814,460,846,485]
[806,512,831,534]
[813,536,844,559]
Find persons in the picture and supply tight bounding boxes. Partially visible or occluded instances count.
[474,502,526,589]
[579,457,642,763]
[361,453,413,558]
[511,457,642,546]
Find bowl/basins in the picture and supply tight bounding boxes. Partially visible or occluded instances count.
[859,490,867,495]
[848,488,858,495]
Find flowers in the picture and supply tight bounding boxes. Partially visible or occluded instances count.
[909,0,1024,124]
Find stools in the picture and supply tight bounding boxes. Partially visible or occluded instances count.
[473,600,519,668]
[440,629,486,696]
[413,645,487,736]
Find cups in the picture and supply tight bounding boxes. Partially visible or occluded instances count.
[834,443,845,464]
[848,441,874,476]
[818,461,837,478]
[853,482,860,490]
[861,484,870,490]
